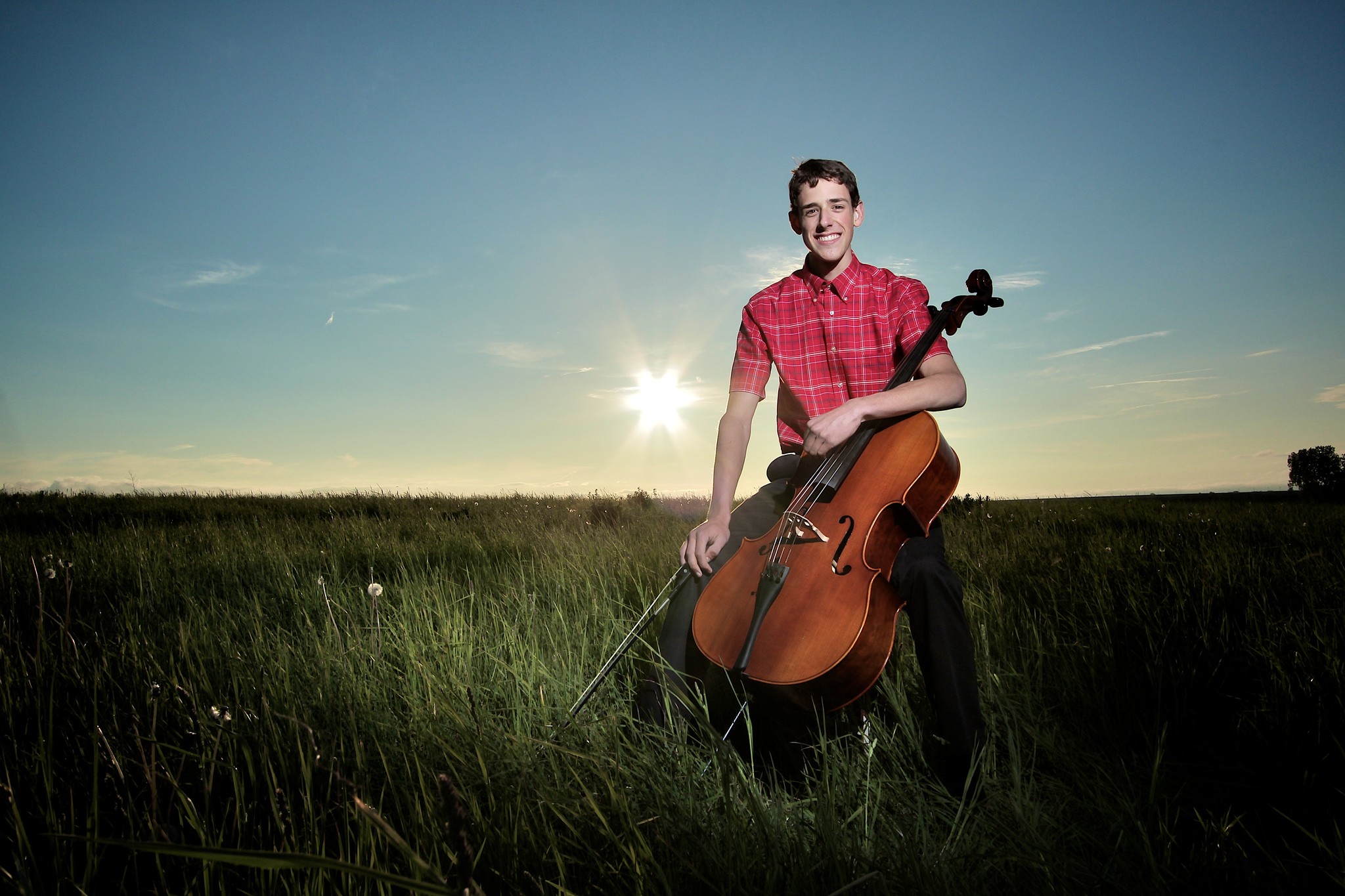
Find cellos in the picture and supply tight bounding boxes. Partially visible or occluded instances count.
[685,266,1005,763]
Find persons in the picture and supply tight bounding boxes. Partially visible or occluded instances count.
[658,159,987,812]
